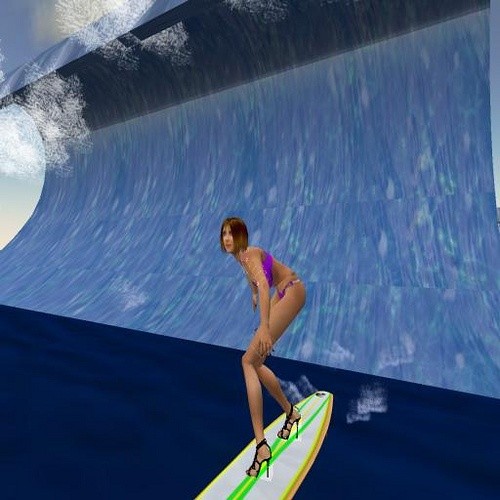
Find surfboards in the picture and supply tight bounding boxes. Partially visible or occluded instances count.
[195,391,333,496]
